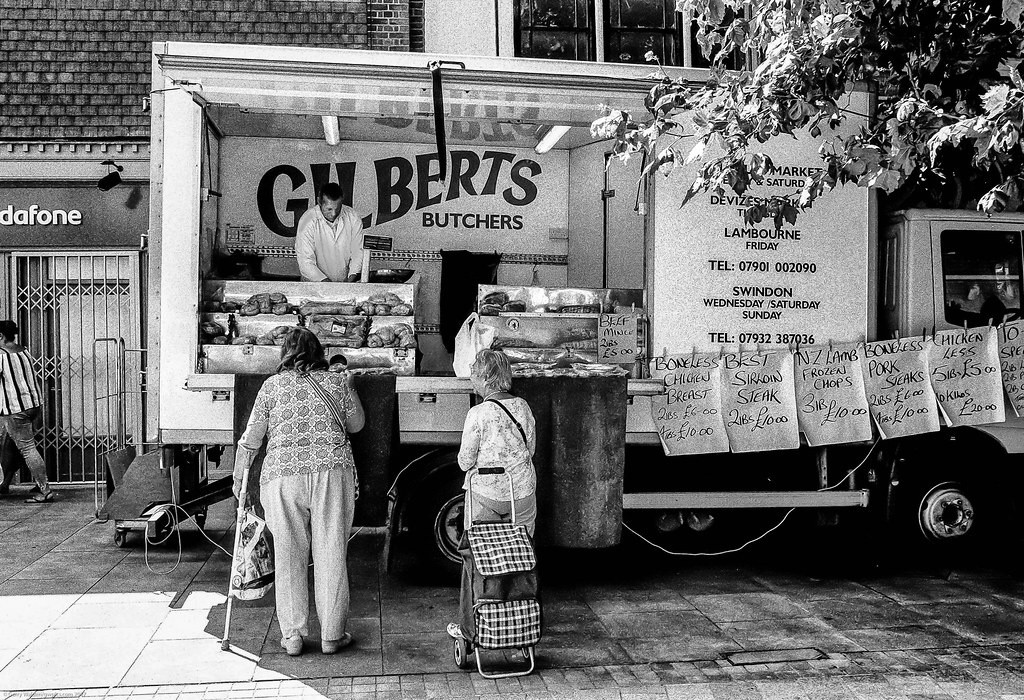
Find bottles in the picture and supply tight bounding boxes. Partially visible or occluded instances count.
[228,313,239,342]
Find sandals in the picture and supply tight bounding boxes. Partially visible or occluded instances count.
[25,488,54,503]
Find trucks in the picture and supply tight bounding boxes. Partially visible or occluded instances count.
[143,40,1023,575]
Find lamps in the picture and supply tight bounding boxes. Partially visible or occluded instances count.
[97,159,123,192]
[533,125,573,155]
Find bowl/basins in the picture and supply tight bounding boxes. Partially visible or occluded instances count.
[368,268,416,284]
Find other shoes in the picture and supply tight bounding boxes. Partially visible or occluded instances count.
[447,621,468,640]
[321,632,352,655]
[280,635,303,655]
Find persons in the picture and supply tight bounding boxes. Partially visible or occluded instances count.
[232,328,365,656]
[445,349,536,644]
[295,182,364,283]
[0,320,54,503]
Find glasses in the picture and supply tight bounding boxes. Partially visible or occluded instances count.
[470,368,477,375]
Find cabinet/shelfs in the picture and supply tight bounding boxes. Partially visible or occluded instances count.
[202,279,415,376]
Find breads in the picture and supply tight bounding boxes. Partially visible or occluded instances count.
[478,294,628,376]
[202,292,418,348]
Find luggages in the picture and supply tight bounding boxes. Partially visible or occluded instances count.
[454,469,542,682]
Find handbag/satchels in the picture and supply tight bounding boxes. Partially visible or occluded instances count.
[230,492,274,601]
